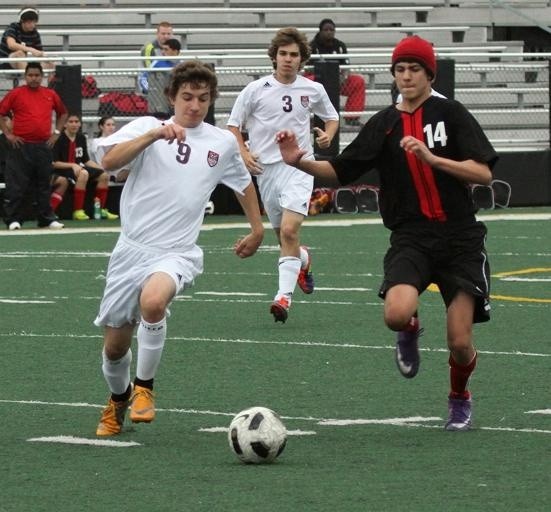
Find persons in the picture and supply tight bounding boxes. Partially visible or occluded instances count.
[274,35,500,431]
[93,61,264,438]
[304,19,365,128]
[226,29,340,325]
[0,6,182,231]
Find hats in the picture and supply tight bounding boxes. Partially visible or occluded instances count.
[18,8,38,23]
[391,36,437,84]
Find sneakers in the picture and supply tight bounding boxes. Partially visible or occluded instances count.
[272,297,290,324]
[8,206,119,230]
[444,390,472,432]
[396,310,423,378]
[296,246,314,294]
[95,383,133,436]
[128,385,155,422]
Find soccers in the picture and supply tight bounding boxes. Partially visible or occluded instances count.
[228,406,289,463]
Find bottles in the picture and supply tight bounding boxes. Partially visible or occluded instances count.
[94,196,100,220]
